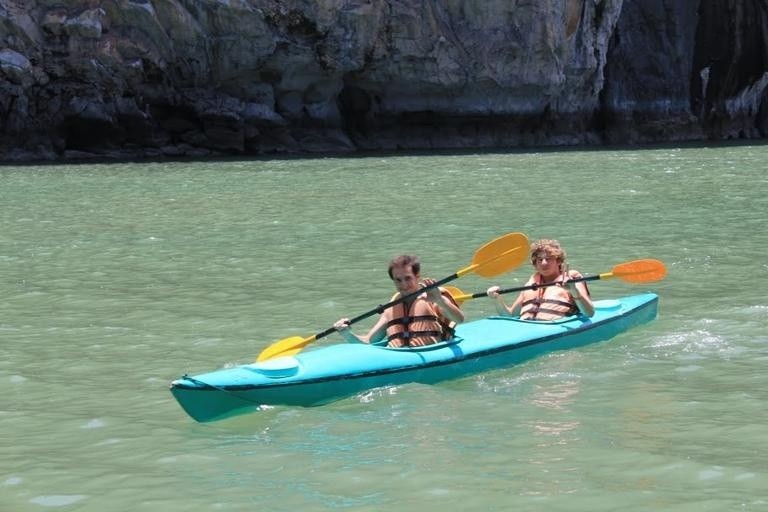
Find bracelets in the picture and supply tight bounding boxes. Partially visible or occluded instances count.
[572,295,584,301]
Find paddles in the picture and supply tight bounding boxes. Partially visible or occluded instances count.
[391,260,667,305]
[257,233,531,364]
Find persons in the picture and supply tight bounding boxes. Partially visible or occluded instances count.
[485,239,597,320]
[332,252,468,349]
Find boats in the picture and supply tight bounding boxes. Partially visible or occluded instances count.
[168,292,660,425]
